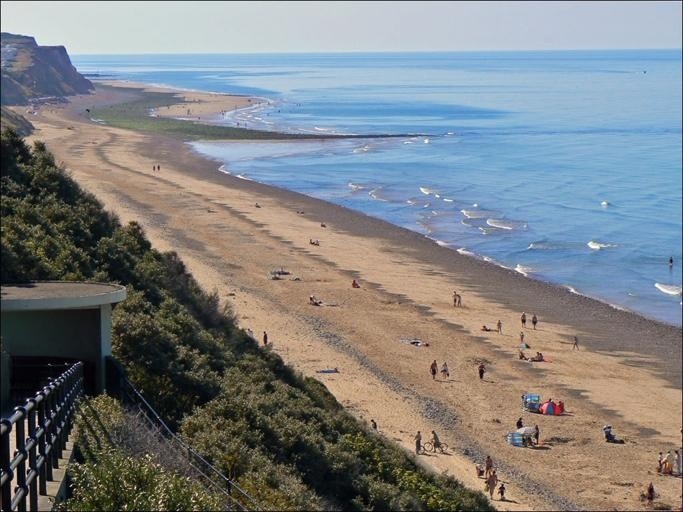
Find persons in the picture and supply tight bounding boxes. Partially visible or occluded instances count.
[530,313,538,330]
[486,470,498,501]
[308,295,316,305]
[474,463,484,478]
[484,455,494,480]
[525,437,533,446]
[534,425,539,445]
[521,437,527,447]
[412,430,423,456]
[547,398,552,403]
[643,481,655,509]
[439,361,448,380]
[451,291,459,307]
[519,311,527,329]
[655,447,681,478]
[603,424,615,443]
[428,359,439,380]
[370,419,377,430]
[350,279,360,288]
[456,295,462,306]
[570,335,579,351]
[261,330,268,346]
[497,483,506,500]
[495,320,502,335]
[428,429,443,454]
[480,325,495,332]
[514,417,523,429]
[517,331,544,362]
[556,400,564,413]
[477,362,487,381]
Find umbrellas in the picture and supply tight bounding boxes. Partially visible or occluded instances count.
[540,402,560,415]
[515,427,538,436]
